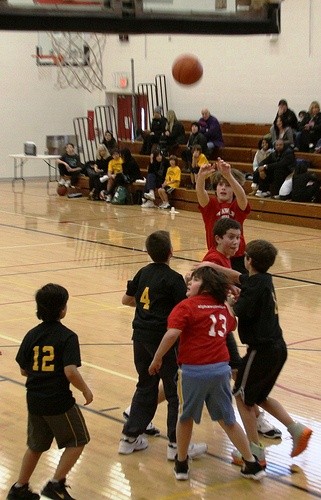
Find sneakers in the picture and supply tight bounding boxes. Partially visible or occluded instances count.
[167,442,208,461]
[6,482,40,500]
[173,453,189,480]
[239,455,267,480]
[41,478,76,500]
[287,420,313,457]
[122,405,160,437]
[118,436,148,454]
[256,412,282,438]
[231,440,266,466]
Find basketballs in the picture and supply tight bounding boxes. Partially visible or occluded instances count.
[172,53,203,86]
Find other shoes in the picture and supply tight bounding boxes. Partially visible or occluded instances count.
[58,179,65,186]
[141,200,154,208]
[65,180,71,188]
[255,190,270,197]
[106,195,112,203]
[249,190,256,195]
[99,175,109,183]
[159,203,170,208]
[144,193,155,200]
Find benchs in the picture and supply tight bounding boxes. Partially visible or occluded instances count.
[60,120,320,229]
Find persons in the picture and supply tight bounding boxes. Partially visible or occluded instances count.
[148,264,267,481]
[3,283,95,500]
[192,238,314,472]
[118,231,208,462]
[247,95,320,207]
[121,218,284,438]
[54,105,221,210]
[194,157,252,290]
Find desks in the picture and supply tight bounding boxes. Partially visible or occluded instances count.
[9,154,62,186]
